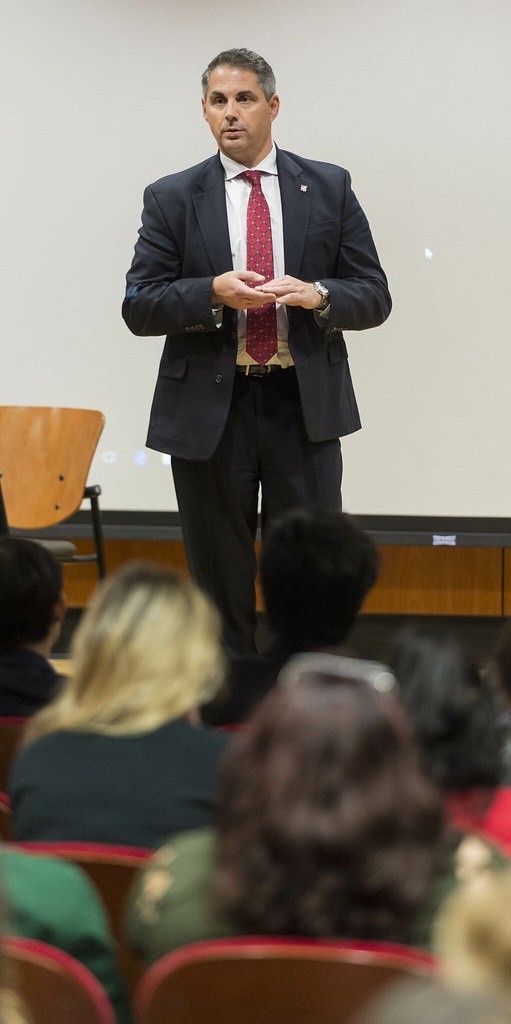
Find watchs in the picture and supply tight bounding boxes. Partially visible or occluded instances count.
[314,281,330,310]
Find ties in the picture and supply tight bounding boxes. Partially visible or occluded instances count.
[237,170,279,366]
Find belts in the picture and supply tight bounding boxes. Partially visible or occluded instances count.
[235,364,296,380]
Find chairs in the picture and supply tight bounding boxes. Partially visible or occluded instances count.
[0,405,109,584]
[0,840,435,1024]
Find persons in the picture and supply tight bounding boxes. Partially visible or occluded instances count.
[116,652,501,969]
[6,566,234,847]
[121,48,392,657]
[0,538,66,716]
[200,513,380,726]
[1,846,129,1024]
[389,623,511,860]
